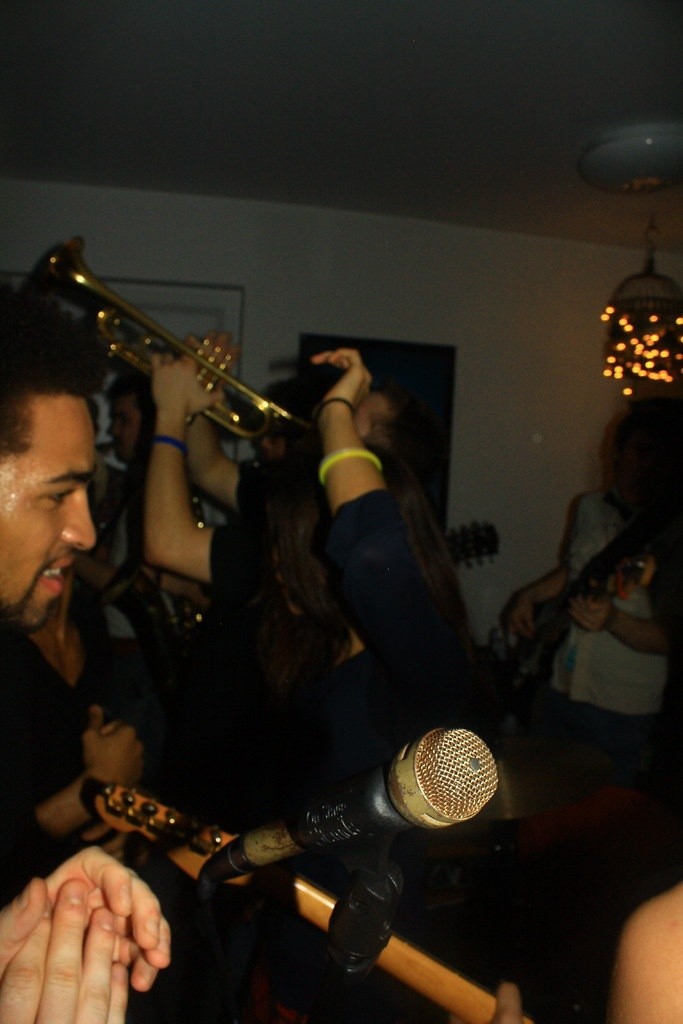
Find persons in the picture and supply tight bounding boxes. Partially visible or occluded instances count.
[0,276,683,1024]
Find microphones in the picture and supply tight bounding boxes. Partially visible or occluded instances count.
[197,726,500,893]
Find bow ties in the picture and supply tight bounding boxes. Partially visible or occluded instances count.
[602,490,633,521]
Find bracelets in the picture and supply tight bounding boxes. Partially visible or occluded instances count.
[314,397,355,418]
[319,448,382,484]
[153,436,188,457]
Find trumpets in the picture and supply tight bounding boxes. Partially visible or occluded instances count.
[22,235,320,444]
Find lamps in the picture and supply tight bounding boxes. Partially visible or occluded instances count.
[578,120,683,195]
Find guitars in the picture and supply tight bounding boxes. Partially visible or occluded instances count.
[93,781,534,1024]
[488,546,657,695]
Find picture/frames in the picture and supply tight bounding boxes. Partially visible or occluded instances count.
[1,270,245,462]
[297,330,458,534]
